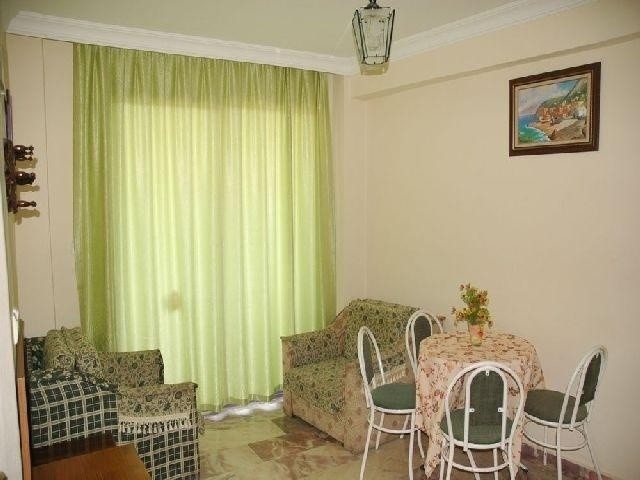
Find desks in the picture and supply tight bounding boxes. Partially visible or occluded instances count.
[410,329,545,480]
[30,438,157,478]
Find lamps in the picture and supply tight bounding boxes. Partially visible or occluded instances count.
[350,0,398,68]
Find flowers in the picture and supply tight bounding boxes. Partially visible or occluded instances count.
[450,281,495,330]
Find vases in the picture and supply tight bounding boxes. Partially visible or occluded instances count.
[467,323,485,347]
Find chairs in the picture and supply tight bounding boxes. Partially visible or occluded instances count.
[518,342,612,480]
[355,324,427,479]
[404,306,448,383]
[279,297,448,456]
[22,324,208,479]
[438,360,528,480]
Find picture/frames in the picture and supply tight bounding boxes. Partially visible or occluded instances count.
[506,59,602,158]
